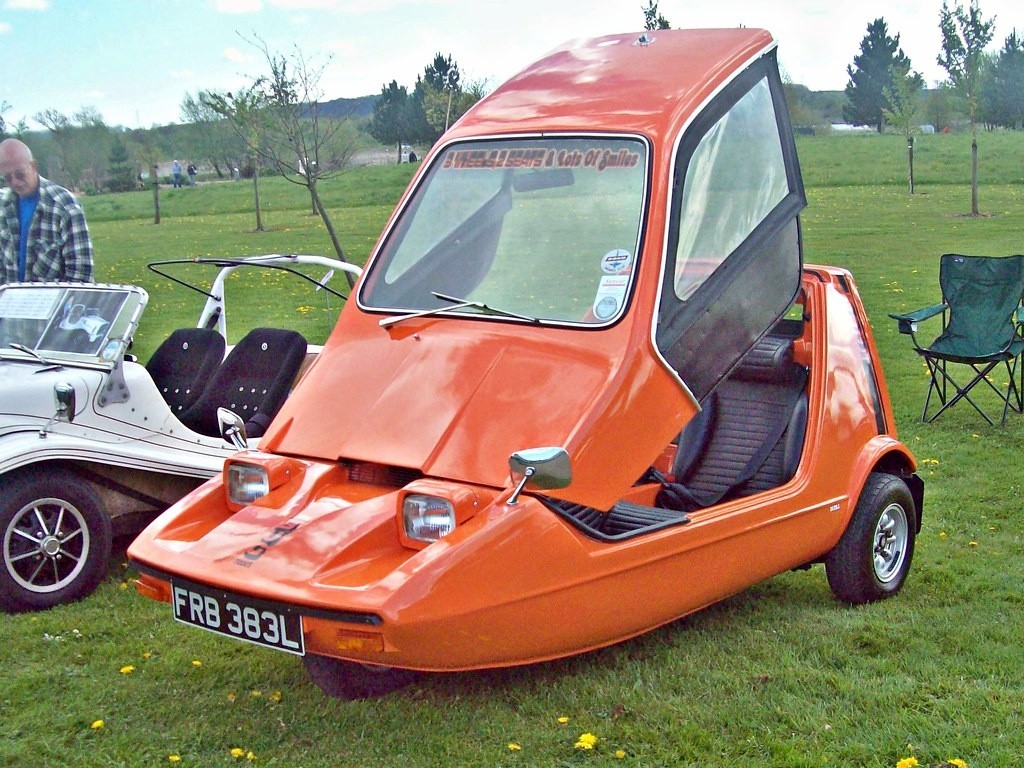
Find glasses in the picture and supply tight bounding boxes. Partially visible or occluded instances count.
[2,162,32,183]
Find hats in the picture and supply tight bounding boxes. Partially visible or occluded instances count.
[174,159,178,163]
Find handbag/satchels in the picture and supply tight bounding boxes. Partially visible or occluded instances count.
[192,165,198,174]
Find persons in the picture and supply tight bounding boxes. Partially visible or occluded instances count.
[172,160,182,188]
[0,138,95,286]
[408,150,417,163]
[402,151,408,163]
[187,161,197,186]
[137,166,145,187]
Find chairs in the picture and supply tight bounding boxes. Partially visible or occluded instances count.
[146,327,227,416]
[175,326,307,441]
[888,254,1024,427]
[657,338,807,511]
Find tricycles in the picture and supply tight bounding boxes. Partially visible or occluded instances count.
[124,28,923,699]
